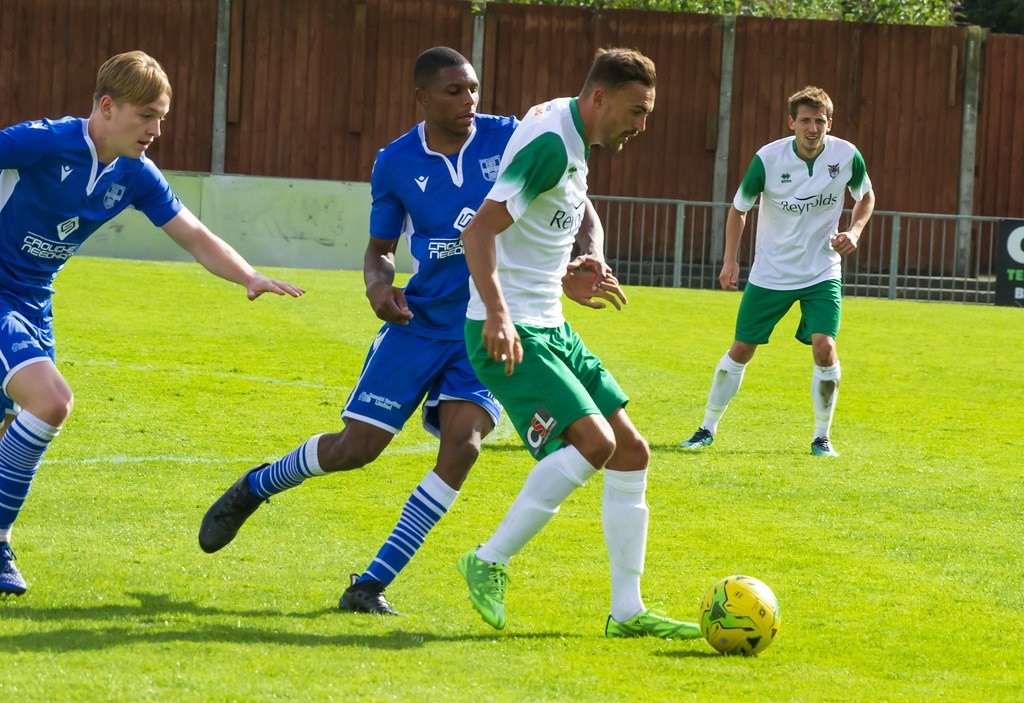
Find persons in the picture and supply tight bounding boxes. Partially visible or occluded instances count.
[196,45,615,614]
[0,49,308,596]
[680,84,877,458]
[457,48,702,641]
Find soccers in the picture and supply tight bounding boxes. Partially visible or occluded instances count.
[697,575,781,656]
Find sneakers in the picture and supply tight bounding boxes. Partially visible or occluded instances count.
[0,538,29,600]
[680,427,715,450]
[605,608,708,642]
[811,437,841,459]
[456,543,510,633]
[196,461,278,557]
[340,577,404,619]
